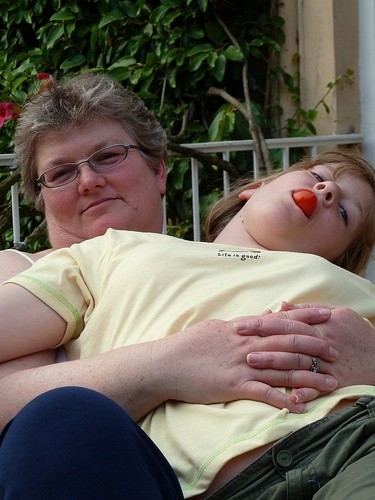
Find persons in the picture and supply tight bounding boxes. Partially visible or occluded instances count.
[0,72,375,500]
[0,145,375,500]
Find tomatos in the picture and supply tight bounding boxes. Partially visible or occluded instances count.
[293,189,318,217]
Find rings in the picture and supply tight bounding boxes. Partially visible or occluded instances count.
[310,357,319,371]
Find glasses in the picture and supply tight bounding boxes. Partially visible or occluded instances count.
[36,143,150,188]
